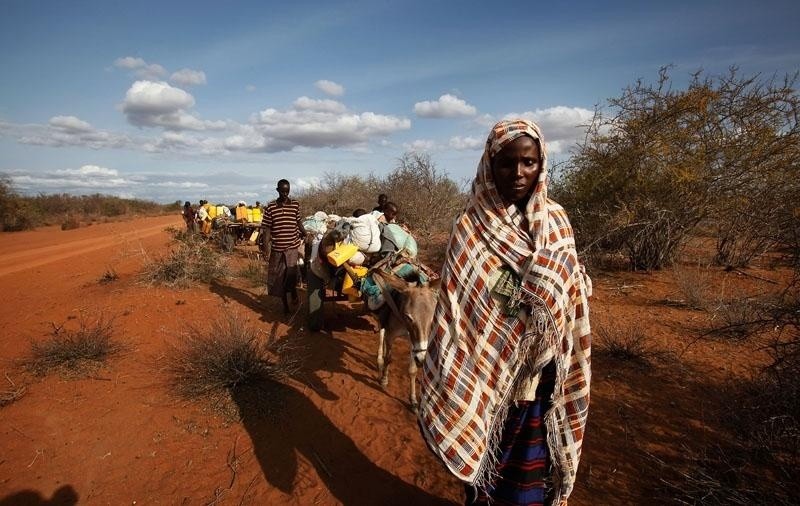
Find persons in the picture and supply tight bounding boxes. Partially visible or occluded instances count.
[260,179,308,313]
[182,200,267,242]
[415,118,594,506]
[352,194,398,224]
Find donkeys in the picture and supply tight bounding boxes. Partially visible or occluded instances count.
[371,249,440,415]
